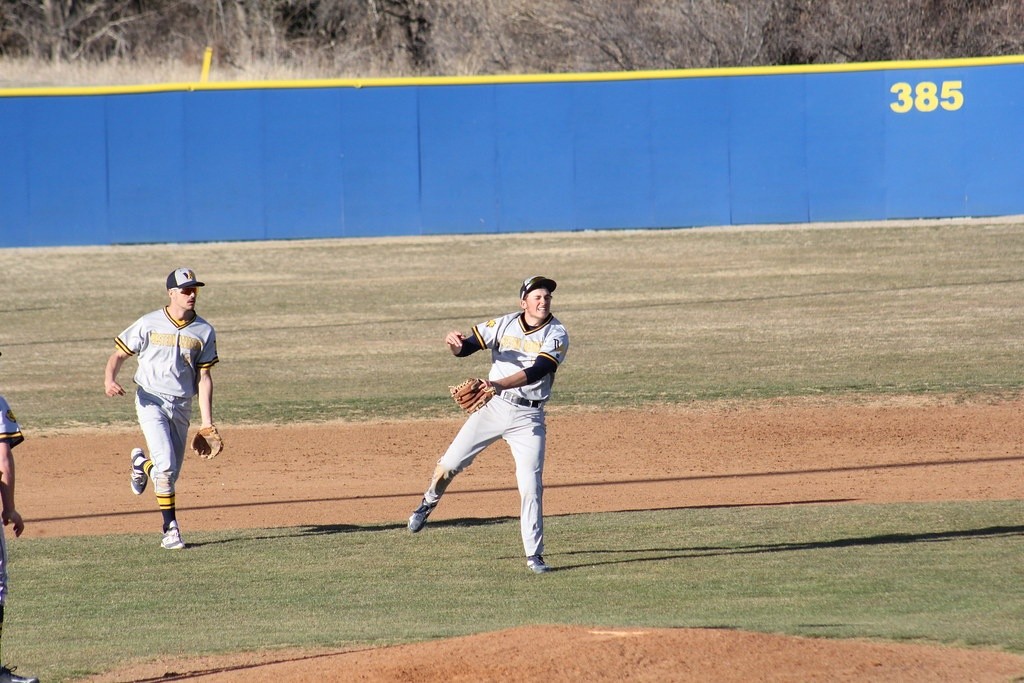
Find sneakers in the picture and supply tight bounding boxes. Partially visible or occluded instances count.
[160,527,186,549]
[131,447,148,495]
[408,498,436,533]
[527,556,550,574]
[0,663,39,683]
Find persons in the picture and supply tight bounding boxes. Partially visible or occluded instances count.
[408,276,568,574]
[105,268,223,549]
[0,352,39,683]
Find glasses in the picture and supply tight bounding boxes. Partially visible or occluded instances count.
[520,276,548,304]
[169,288,196,296]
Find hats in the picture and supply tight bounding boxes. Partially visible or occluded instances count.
[166,268,205,291]
[519,276,557,303]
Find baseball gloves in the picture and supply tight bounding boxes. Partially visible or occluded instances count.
[449,377,497,415]
[190,425,224,461]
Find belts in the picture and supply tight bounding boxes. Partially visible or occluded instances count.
[498,390,539,408]
